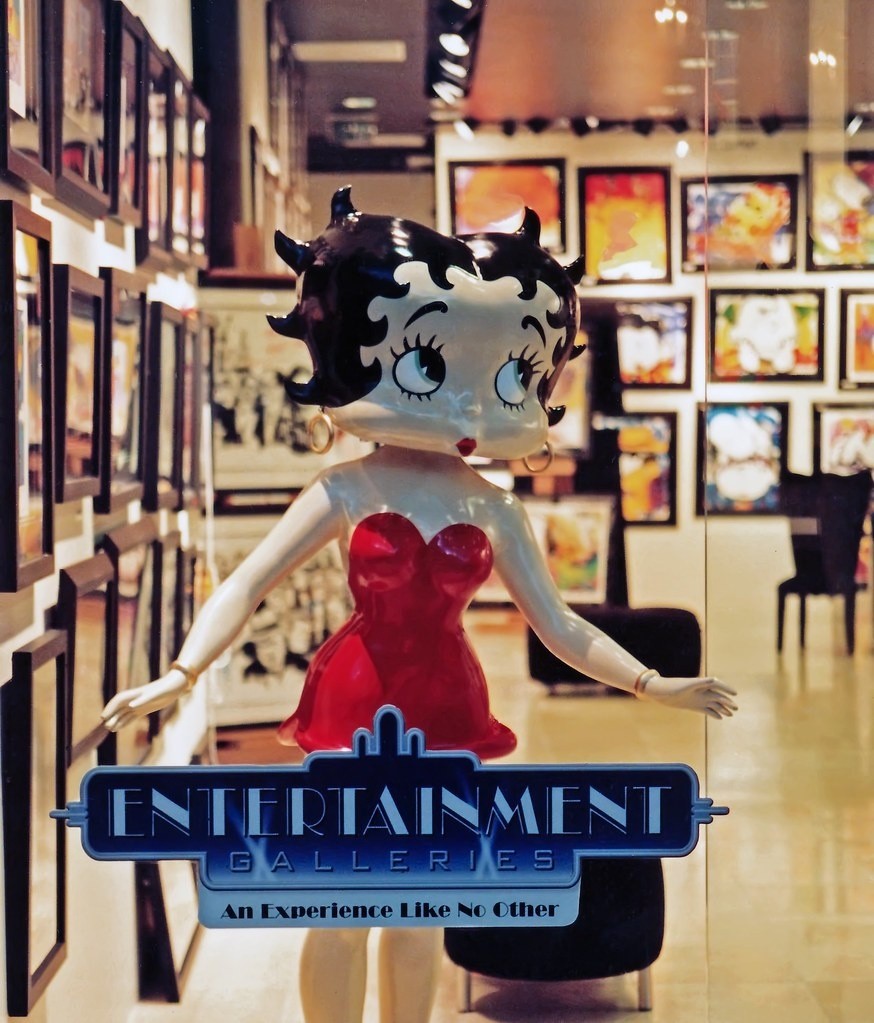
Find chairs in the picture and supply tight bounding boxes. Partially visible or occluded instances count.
[778,466,874,655]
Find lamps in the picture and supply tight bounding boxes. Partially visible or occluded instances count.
[439,3,484,55]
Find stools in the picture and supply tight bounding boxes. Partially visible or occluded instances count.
[527,603,700,696]
[444,856,666,1012]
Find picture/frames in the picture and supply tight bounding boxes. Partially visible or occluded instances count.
[211,505,358,730]
[0,200,214,592]
[696,401,789,515]
[6,515,207,1017]
[249,0,313,273]
[618,412,676,526]
[449,158,567,254]
[195,276,380,494]
[840,288,874,389]
[577,166,672,284]
[813,401,874,480]
[803,149,873,271]
[544,327,599,453]
[134,727,213,1003]
[581,297,694,389]
[1,0,212,274]
[465,464,522,608]
[679,175,800,272]
[517,492,618,605]
[707,287,825,381]
